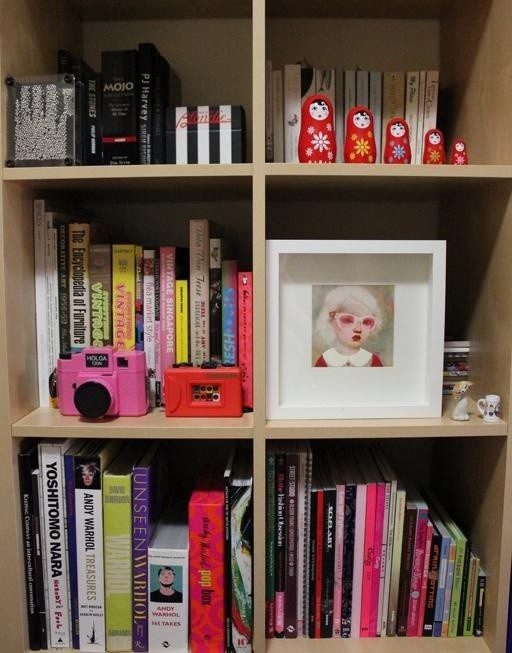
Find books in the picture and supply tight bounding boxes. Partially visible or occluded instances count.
[274,64,438,164]
[266,442,485,637]
[34,199,251,410]
[17,439,253,652]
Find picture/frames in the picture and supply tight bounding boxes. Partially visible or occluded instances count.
[267,238,446,421]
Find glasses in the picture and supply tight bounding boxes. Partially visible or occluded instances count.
[329,311,378,332]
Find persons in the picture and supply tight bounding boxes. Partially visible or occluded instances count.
[314,285,384,367]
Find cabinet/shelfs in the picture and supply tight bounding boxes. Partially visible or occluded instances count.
[0,0,511,653]
